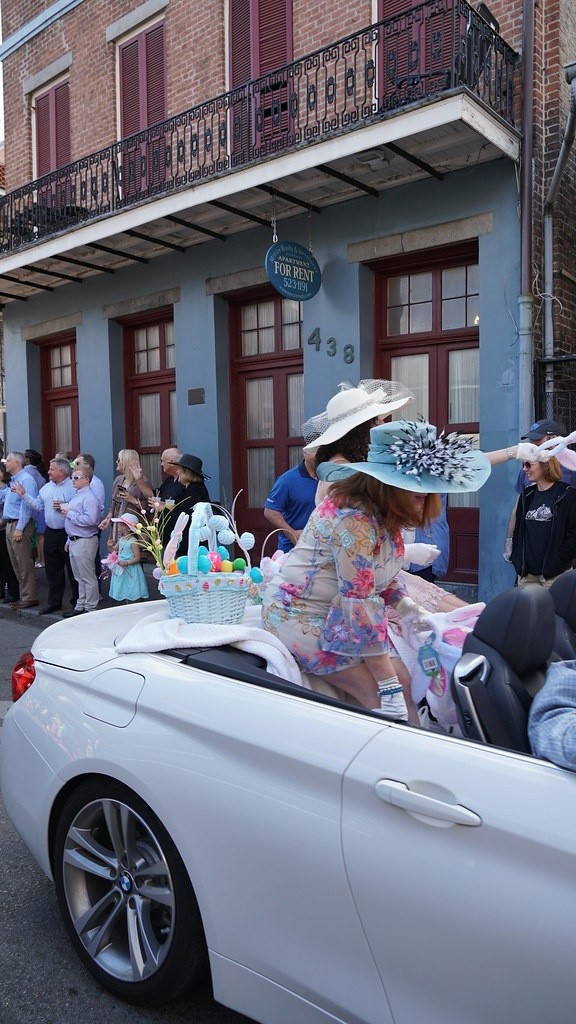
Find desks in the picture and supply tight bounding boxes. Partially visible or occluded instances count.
[17,205,84,237]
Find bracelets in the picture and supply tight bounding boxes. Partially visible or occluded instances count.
[507,448,512,460]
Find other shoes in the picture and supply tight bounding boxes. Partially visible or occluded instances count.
[39,605,62,615]
[12,601,38,608]
[63,610,84,618]
[4,595,19,603]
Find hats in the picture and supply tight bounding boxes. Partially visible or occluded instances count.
[111,513,138,532]
[317,411,491,492]
[168,454,212,480]
[301,379,415,456]
[521,420,560,440]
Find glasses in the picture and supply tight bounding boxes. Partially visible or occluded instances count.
[71,476,86,480]
[522,462,541,468]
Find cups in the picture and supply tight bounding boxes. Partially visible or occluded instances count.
[52,497,59,508]
[153,498,161,502]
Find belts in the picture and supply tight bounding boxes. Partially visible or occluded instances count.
[69,536,82,541]
[7,519,19,523]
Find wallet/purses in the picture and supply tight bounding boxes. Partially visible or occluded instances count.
[113,485,125,503]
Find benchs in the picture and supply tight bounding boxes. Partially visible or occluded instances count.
[374,3,500,116]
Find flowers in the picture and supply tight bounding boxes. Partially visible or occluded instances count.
[120,498,190,575]
[99,551,133,579]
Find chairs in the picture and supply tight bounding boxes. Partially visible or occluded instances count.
[450,583,556,753]
[540,568,576,666]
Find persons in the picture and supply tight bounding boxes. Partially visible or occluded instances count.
[527,585,576,771]
[0,447,212,617]
[262,419,492,725]
[107,513,150,604]
[400,493,451,584]
[510,451,576,588]
[503,419,576,564]
[264,432,319,552]
[303,379,550,615]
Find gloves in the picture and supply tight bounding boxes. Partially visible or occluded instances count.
[396,598,429,627]
[403,542,442,566]
[377,676,409,720]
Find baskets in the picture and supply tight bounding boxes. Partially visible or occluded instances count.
[158,572,251,624]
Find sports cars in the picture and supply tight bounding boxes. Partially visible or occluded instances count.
[0,599,575,1024]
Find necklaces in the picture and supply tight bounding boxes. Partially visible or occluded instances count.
[121,538,129,550]
[313,475,316,478]
[377,684,403,698]
